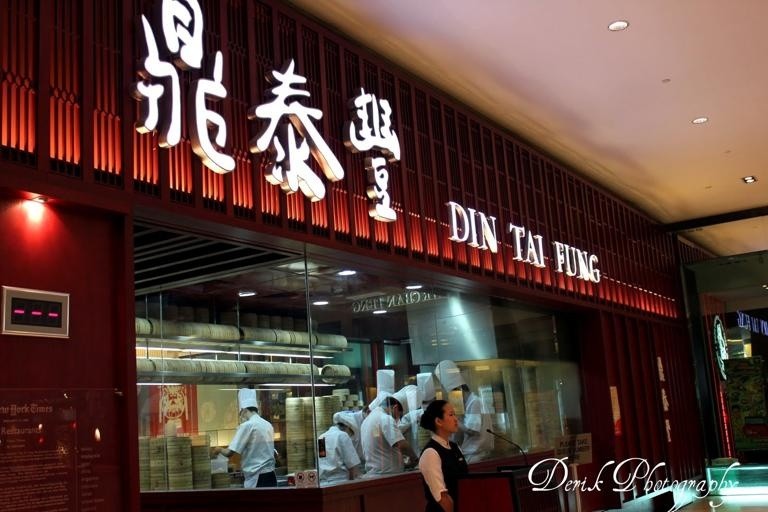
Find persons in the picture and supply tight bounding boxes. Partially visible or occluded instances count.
[214,407,277,487]
[316,384,494,486]
[418,400,469,512]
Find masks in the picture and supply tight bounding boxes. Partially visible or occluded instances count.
[239,412,247,423]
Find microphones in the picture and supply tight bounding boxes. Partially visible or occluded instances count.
[487,429,528,465]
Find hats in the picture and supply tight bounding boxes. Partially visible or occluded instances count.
[238,388,259,413]
[332,360,467,435]
